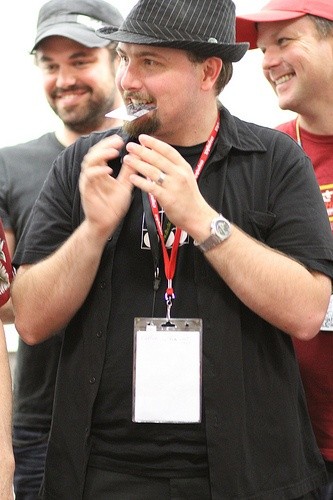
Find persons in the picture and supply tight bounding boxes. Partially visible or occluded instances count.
[0,218,15,500]
[11,0,333,500]
[235,0,333,500]
[0,1,124,500]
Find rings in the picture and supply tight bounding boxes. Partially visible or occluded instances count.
[157,173,166,185]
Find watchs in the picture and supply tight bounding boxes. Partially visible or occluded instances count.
[193,212,231,253]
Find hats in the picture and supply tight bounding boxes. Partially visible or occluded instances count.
[27,0,123,54]
[95,0,249,62]
[235,0,332,50]
